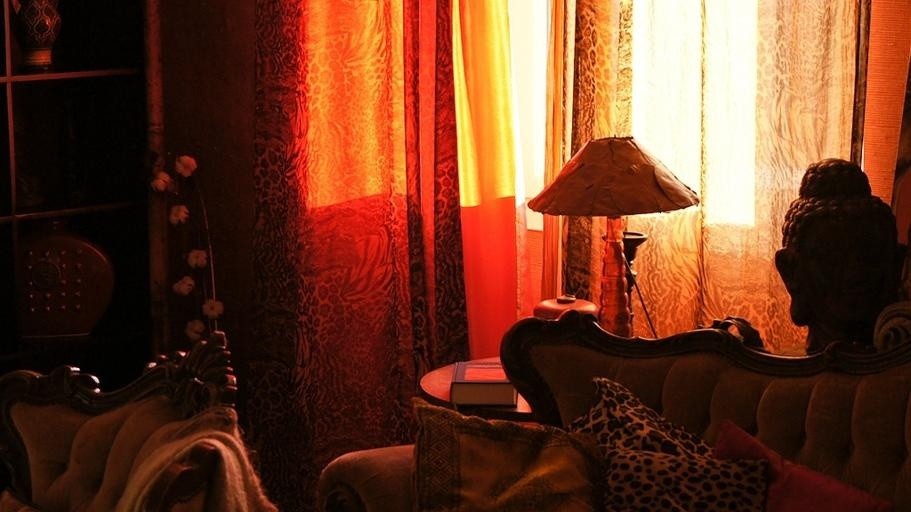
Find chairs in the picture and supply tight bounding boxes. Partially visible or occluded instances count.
[1,330,240,512]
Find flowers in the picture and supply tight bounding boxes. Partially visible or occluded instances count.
[137,146,226,342]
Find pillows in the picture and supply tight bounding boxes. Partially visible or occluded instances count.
[715,416,760,463]
[574,375,773,509]
[415,398,594,509]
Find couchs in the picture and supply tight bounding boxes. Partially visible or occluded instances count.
[312,307,911,512]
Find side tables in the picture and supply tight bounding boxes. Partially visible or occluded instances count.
[417,355,534,424]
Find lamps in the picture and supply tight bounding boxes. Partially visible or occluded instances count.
[527,137,701,337]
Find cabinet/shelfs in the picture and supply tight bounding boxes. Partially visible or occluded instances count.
[1,1,149,369]
